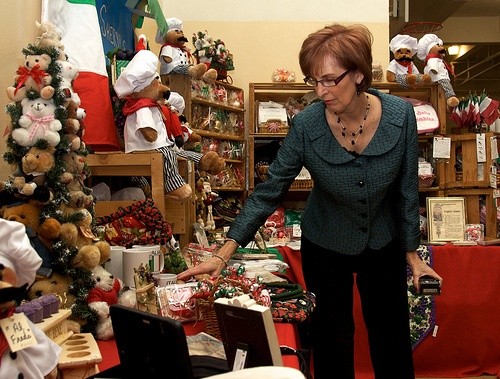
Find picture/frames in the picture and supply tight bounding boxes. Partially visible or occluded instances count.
[426,195,466,242]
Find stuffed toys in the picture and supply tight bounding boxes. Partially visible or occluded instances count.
[386,34,430,89]
[0,18,244,379]
[416,33,459,107]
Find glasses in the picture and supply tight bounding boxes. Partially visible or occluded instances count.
[303,68,351,87]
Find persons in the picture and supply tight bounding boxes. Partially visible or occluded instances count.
[175,24,442,379]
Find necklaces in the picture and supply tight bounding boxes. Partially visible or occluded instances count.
[334,96,371,146]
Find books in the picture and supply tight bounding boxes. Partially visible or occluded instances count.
[214,298,282,371]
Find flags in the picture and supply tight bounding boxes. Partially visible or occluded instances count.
[38,0,135,150]
[447,88,500,130]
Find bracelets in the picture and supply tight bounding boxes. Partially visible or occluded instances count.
[212,255,227,266]
[224,239,238,244]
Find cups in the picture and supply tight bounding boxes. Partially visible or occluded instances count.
[152,273,178,287]
[104,244,165,287]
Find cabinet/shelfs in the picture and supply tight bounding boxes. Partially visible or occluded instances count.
[444,133,500,246]
[86,150,196,248]
[163,73,248,223]
[248,82,443,218]
